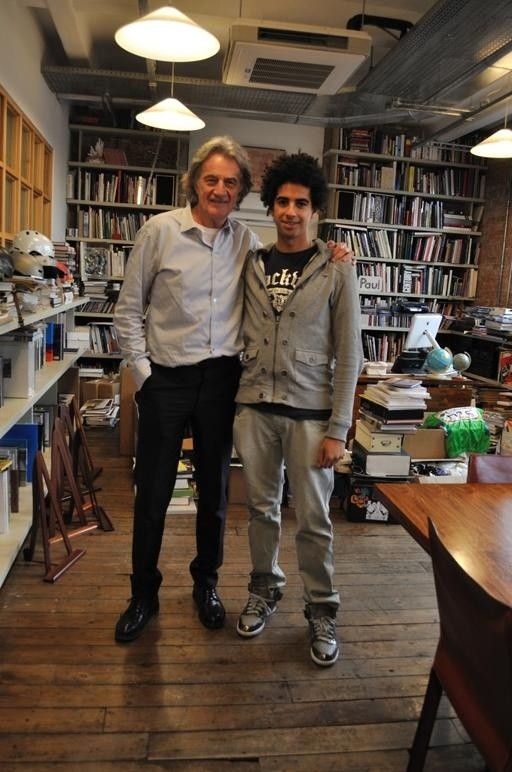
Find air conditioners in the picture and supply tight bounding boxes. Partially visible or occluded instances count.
[221,14,372,99]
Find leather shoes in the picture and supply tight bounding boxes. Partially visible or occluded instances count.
[115,597,159,644]
[193,585,226,630]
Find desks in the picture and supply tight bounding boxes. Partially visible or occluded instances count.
[374,474,512,705]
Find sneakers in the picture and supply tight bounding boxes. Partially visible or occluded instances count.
[236,583,283,638]
[303,606,339,666]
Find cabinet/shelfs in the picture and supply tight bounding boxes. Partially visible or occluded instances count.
[318,116,486,364]
[454,326,512,387]
[0,80,56,265]
[62,95,191,367]
[0,282,95,593]
[348,373,474,428]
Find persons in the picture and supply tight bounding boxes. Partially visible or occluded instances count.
[107,135,353,647]
[234,150,367,667]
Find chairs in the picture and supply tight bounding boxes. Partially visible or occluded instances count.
[399,512,512,772]
[462,449,512,486]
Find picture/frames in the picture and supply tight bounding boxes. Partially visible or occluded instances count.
[237,143,289,196]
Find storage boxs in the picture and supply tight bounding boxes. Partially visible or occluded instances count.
[353,419,406,452]
[399,426,449,459]
[79,377,122,407]
[344,471,411,525]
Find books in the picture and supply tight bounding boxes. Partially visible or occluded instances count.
[50,236,82,298]
[73,127,160,432]
[1,311,74,534]
[163,451,203,515]
[323,122,512,483]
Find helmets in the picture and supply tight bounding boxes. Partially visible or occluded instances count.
[8,230,55,260]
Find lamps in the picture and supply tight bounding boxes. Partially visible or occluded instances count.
[128,63,209,136]
[468,75,512,165]
[105,0,224,67]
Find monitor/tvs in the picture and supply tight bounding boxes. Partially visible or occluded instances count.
[405,313,442,349]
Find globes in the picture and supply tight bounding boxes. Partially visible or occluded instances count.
[426,347,453,375]
[454,352,471,380]
